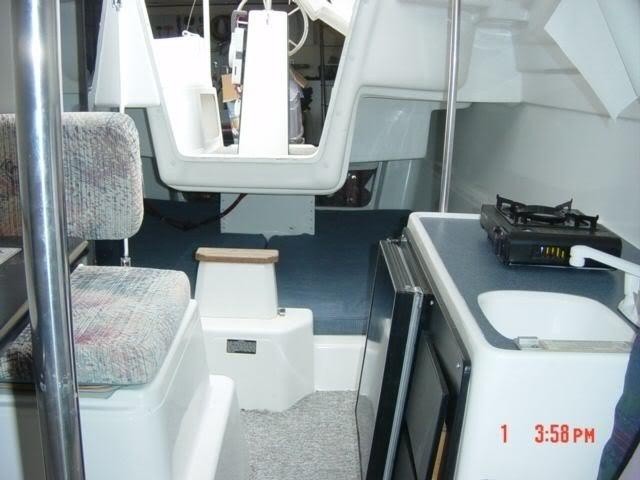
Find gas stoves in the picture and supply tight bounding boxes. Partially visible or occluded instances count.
[480,193,622,273]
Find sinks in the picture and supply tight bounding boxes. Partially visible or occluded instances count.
[477,289,635,341]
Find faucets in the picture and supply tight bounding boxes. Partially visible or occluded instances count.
[568,244,636,278]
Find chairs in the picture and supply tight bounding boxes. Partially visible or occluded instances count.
[0,112,199,480]
